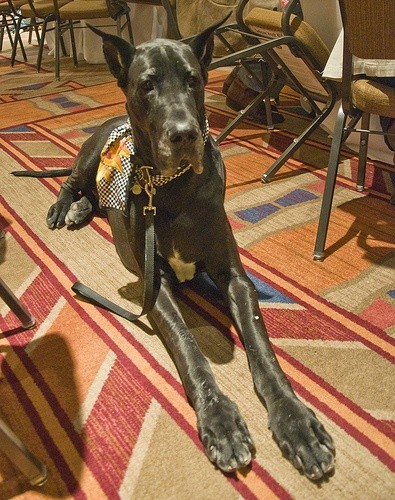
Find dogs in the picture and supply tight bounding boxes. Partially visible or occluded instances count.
[9,9,339,483]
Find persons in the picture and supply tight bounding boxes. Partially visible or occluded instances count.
[322,26,395,205]
[176,0,284,125]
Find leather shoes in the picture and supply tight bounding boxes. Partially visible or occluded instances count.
[223,96,284,123]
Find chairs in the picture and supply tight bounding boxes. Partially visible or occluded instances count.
[173,0,341,181]
[0,0,135,81]
[312,0,395,259]
